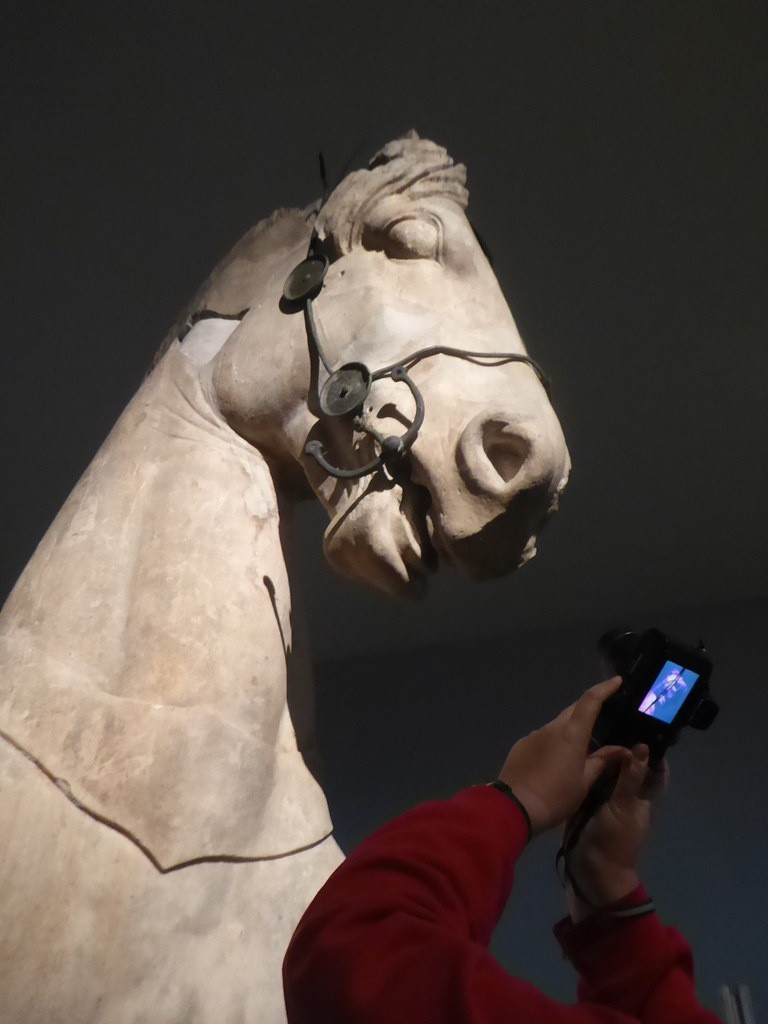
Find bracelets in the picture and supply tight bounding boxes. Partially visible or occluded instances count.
[487,779,532,841]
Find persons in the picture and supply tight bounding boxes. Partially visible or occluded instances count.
[282,675,722,1024]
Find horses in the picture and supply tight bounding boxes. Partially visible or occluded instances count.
[0,128,574,1024]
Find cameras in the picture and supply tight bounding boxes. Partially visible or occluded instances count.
[586,626,719,767]
